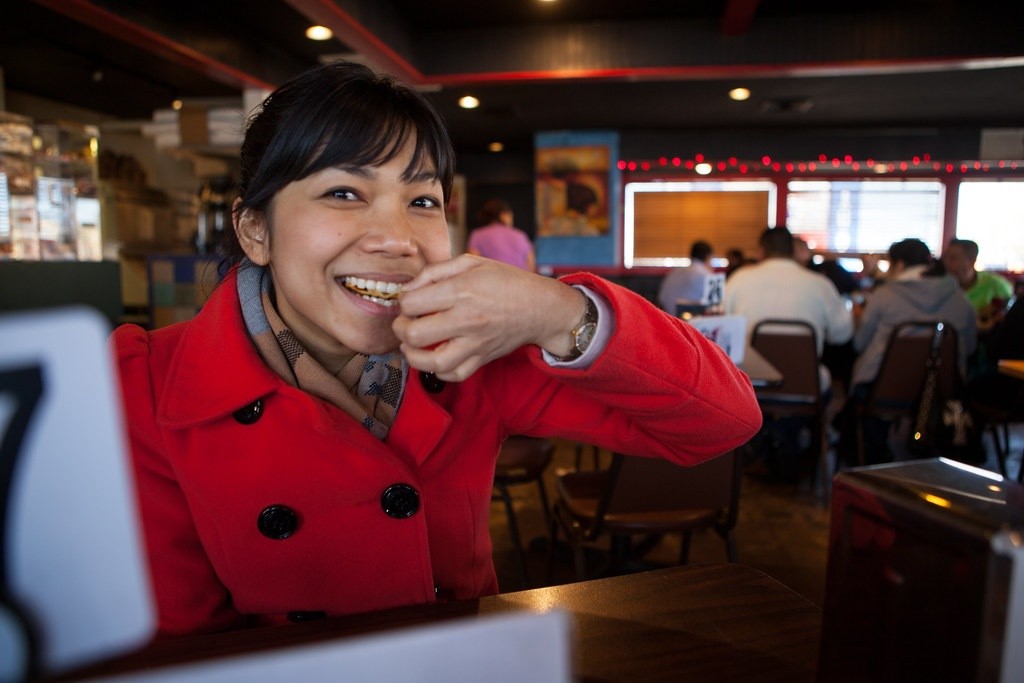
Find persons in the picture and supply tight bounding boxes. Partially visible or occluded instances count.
[941,237,1013,333]
[967,291,1023,484]
[727,247,745,279]
[658,239,718,317]
[854,237,978,414]
[466,200,537,272]
[722,226,855,412]
[107,57,765,648]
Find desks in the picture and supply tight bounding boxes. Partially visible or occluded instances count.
[52,563,820,683]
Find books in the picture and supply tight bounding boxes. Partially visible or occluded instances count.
[138,107,246,151]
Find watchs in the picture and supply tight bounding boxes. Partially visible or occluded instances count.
[555,291,599,364]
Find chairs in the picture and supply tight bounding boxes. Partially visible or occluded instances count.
[747,320,835,490]
[846,320,956,468]
[494,438,559,588]
[552,447,741,581]
